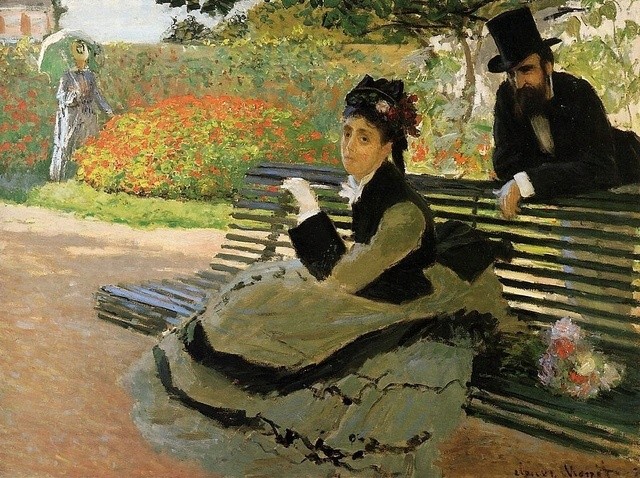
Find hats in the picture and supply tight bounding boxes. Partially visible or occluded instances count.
[485,6,562,73]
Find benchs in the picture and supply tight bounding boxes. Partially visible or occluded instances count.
[92,162,640,466]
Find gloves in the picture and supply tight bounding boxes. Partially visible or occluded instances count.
[282,176,321,226]
